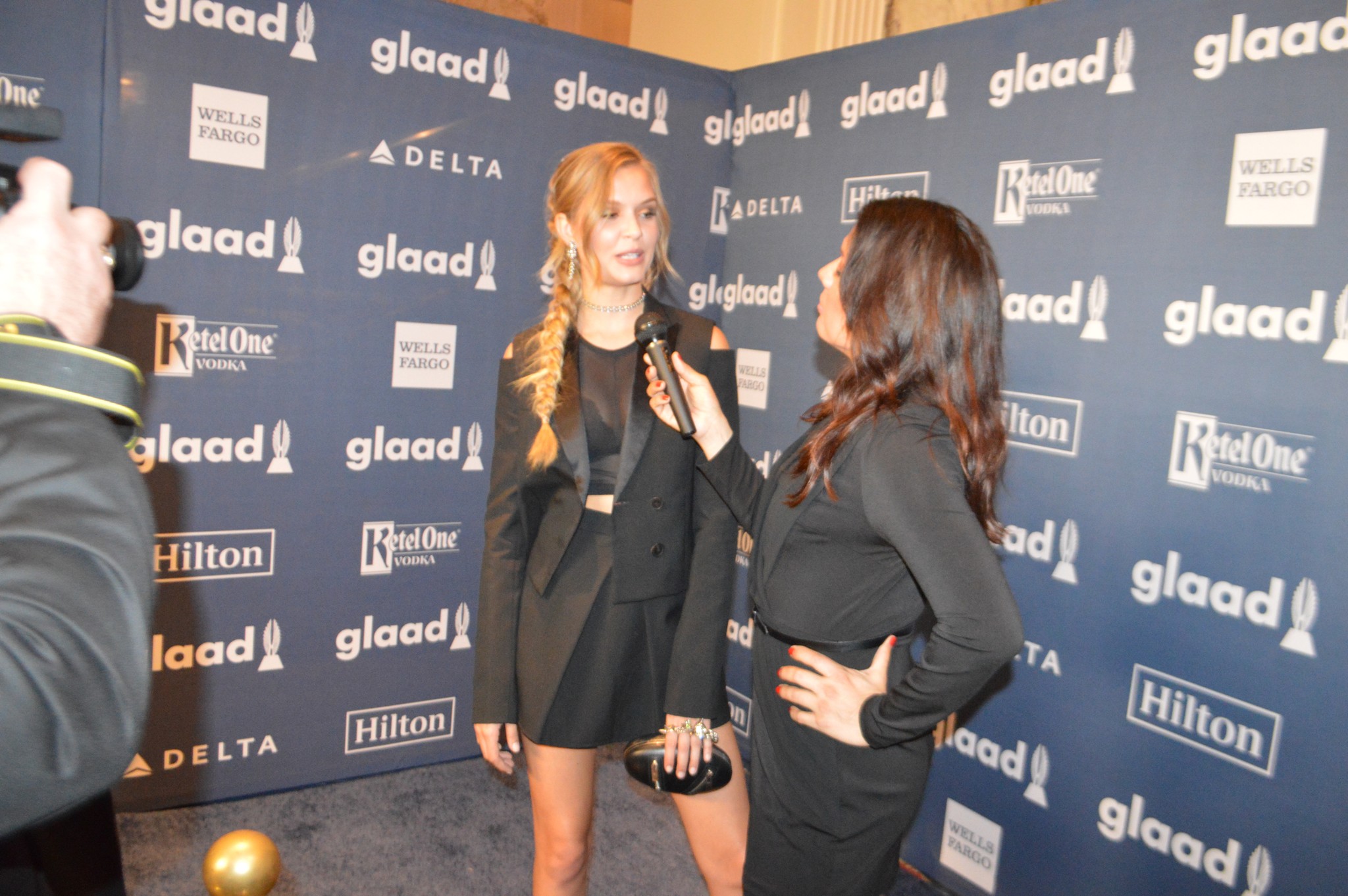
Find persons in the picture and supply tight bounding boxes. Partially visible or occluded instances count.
[638,195,1018,896]
[466,135,751,896]
[0,155,163,894]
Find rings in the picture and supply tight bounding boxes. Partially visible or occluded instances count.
[658,716,718,743]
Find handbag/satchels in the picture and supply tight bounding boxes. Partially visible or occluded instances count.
[621,733,732,798]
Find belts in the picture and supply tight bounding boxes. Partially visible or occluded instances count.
[748,609,917,653]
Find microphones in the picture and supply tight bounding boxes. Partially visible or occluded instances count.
[633,311,697,439]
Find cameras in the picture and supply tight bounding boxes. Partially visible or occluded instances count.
[0,99,146,293]
